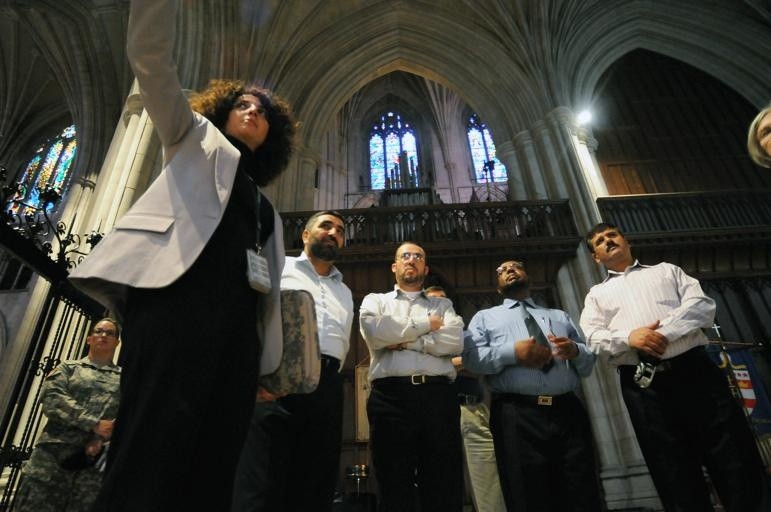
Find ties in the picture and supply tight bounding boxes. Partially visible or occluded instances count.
[517,300,555,374]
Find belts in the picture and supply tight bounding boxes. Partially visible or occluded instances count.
[492,391,573,407]
[375,376,451,386]
[320,356,341,370]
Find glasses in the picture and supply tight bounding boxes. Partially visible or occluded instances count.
[496,261,529,274]
[395,252,425,263]
